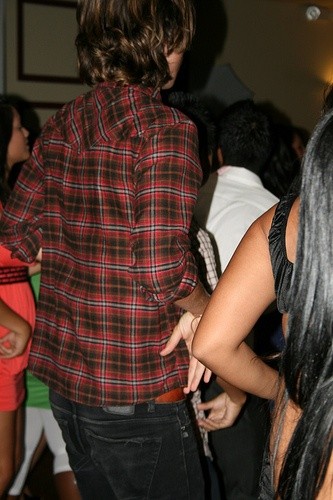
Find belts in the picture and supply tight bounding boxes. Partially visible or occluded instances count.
[154,387,186,404]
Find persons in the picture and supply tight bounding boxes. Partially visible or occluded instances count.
[0,0,246,500]
[0,84,333,500]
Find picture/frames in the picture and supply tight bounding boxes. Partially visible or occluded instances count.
[30,102,67,130]
[16,0,88,85]
